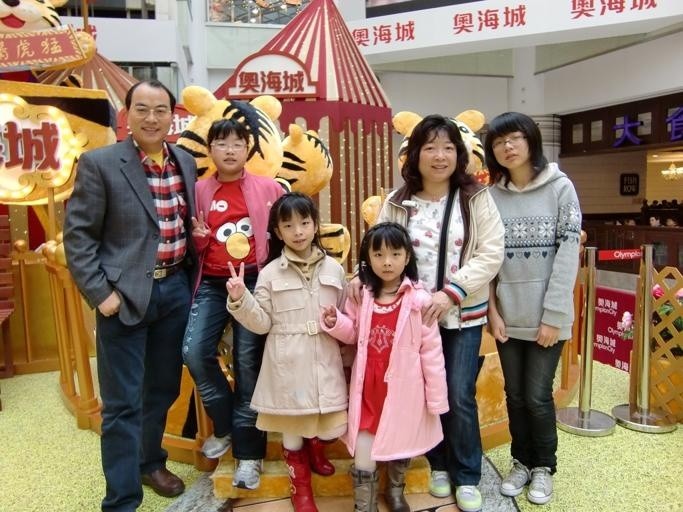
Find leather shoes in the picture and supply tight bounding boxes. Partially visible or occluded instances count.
[141,466,184,498]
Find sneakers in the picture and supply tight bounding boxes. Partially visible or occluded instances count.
[231,458,264,491]
[526,466,553,506]
[454,485,482,512]
[499,460,529,497]
[200,432,233,460]
[430,471,451,498]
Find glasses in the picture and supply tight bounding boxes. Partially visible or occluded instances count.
[491,135,525,150]
[209,141,249,152]
[130,105,174,120]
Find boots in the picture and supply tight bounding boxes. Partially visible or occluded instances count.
[307,437,334,476]
[384,459,411,512]
[348,465,379,512]
[282,444,318,512]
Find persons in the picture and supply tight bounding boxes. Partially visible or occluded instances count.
[321,222,450,512]
[377,114,505,512]
[225,191,349,511]
[649,215,663,226]
[176,119,286,491]
[478,111,585,506]
[64,78,203,512]
[623,218,638,225]
[663,217,678,226]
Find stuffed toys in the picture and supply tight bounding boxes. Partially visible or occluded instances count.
[274,123,352,261]
[167,82,294,196]
[361,107,487,241]
